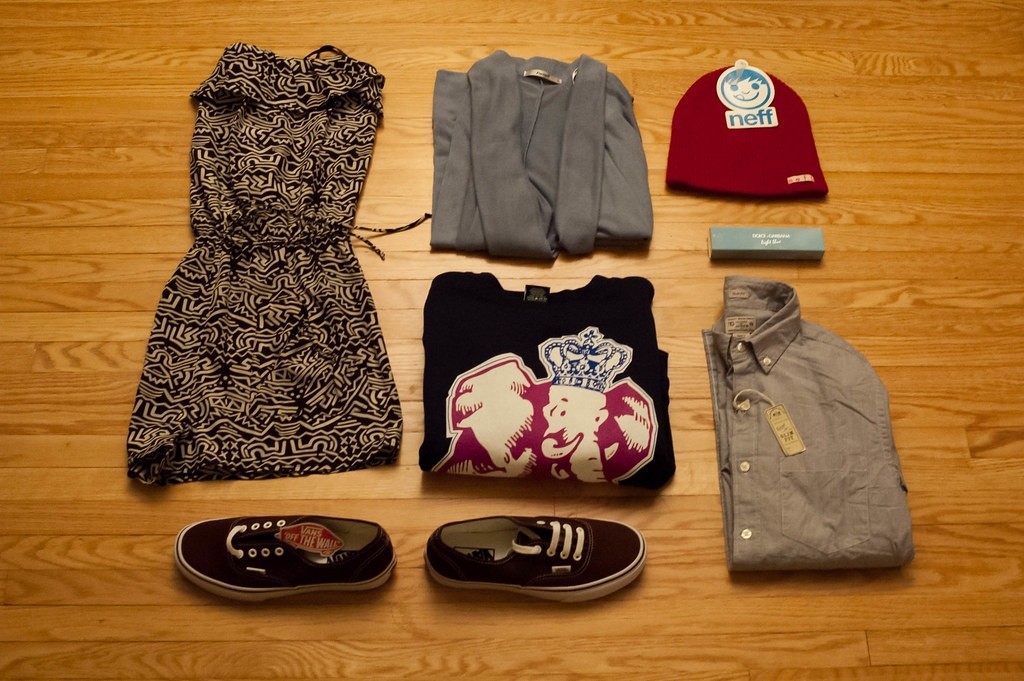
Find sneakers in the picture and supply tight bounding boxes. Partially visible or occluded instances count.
[426,514,649,602]
[172,514,394,602]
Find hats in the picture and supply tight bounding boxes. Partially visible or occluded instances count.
[666,60,830,201]
